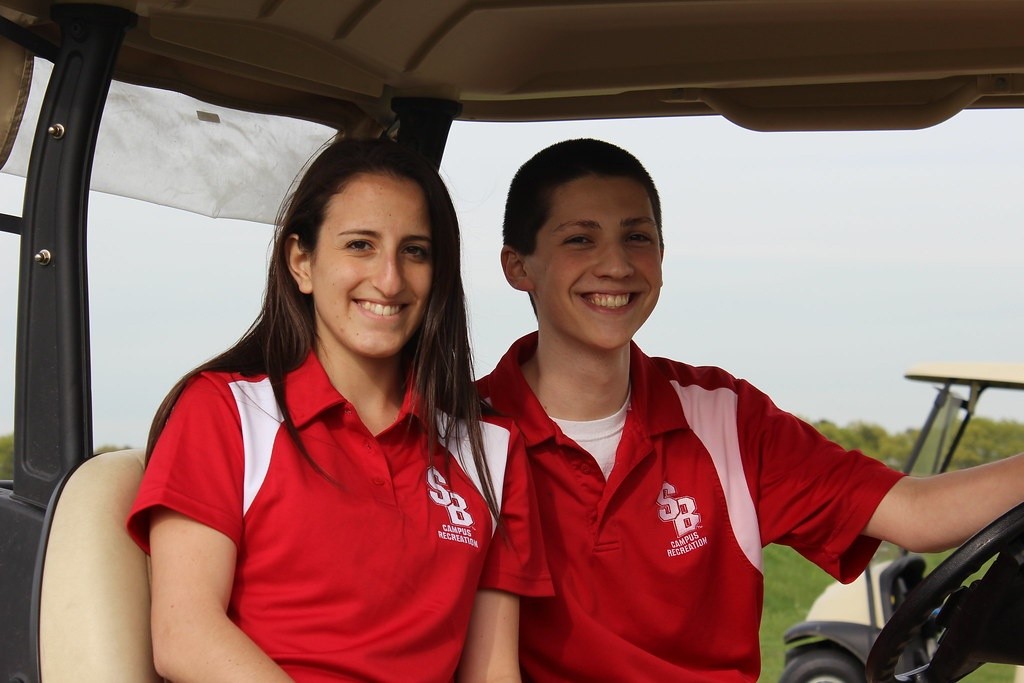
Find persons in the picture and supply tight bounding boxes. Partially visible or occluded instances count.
[473,137,1024,683]
[123,128,555,682]
